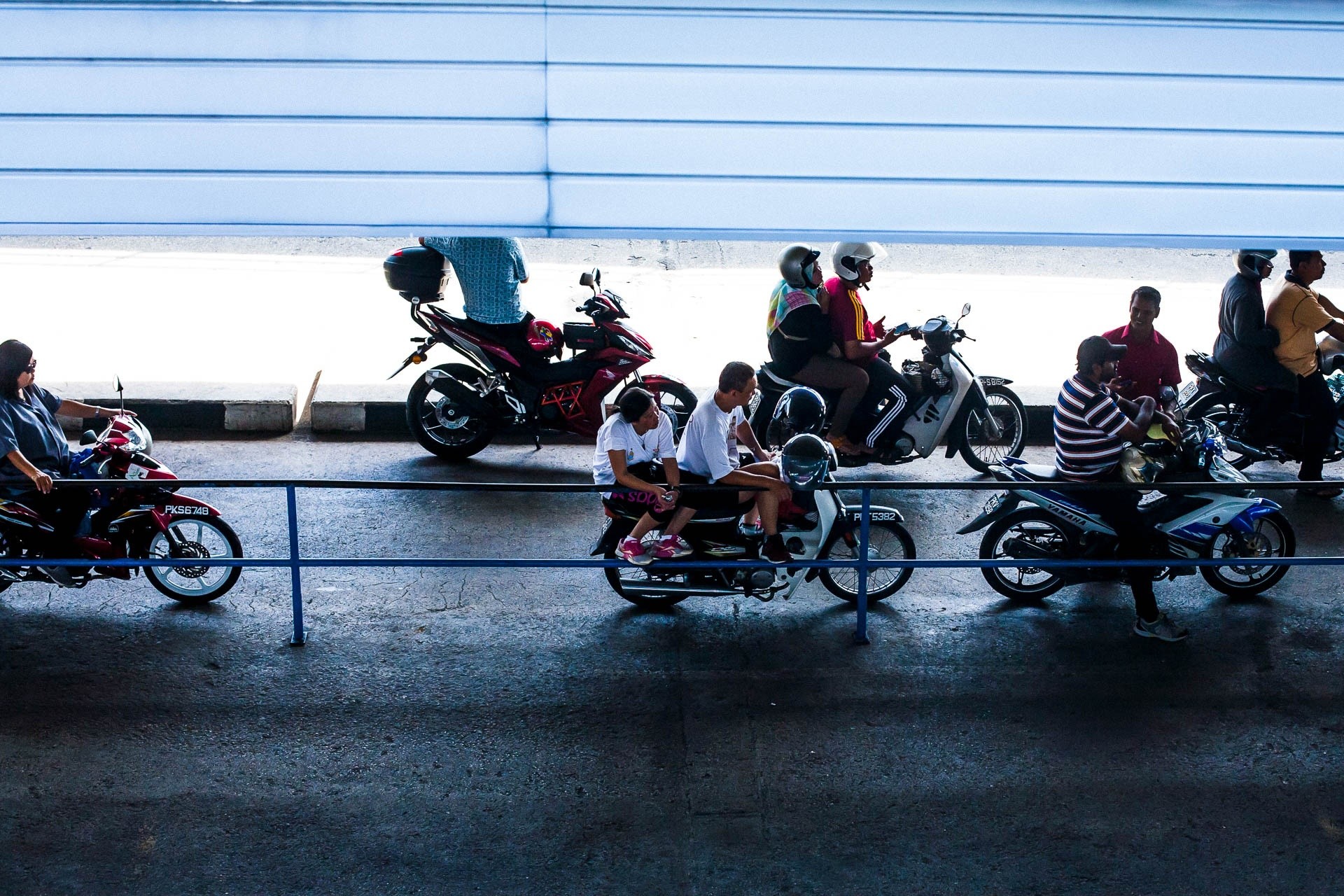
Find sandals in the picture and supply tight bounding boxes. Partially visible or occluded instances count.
[825,433,861,456]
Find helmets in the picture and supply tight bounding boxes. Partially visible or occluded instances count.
[777,243,822,289]
[1237,248,1278,280]
[1138,422,1178,457]
[1317,334,1344,376]
[830,242,875,291]
[781,433,829,491]
[773,386,827,434]
[528,319,565,361]
[1121,447,1159,494]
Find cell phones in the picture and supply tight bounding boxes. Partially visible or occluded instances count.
[1120,380,1132,385]
[888,322,912,334]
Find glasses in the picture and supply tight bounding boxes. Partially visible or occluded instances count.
[26,360,37,374]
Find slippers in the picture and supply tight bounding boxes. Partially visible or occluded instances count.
[861,447,895,460]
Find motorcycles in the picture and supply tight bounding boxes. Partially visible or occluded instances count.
[956,386,1295,602]
[0,372,242,605]
[589,424,916,608]
[743,304,1028,474]
[381,246,697,476]
[1176,350,1344,473]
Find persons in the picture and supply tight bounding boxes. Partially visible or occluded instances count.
[1211,248,1300,450]
[0,338,137,587]
[1051,335,1194,641]
[674,361,796,564]
[1265,248,1344,498]
[761,239,871,460]
[592,387,709,565]
[819,242,917,463]
[417,236,536,345]
[1102,284,1182,422]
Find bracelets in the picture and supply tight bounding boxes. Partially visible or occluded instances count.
[94,405,102,419]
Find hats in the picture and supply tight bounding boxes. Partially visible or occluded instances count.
[1075,335,1128,371]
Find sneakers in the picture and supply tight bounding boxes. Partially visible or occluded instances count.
[37,564,76,588]
[740,519,765,534]
[614,538,652,566]
[759,533,794,564]
[1298,472,1324,487]
[649,534,693,557]
[1133,612,1188,642]
